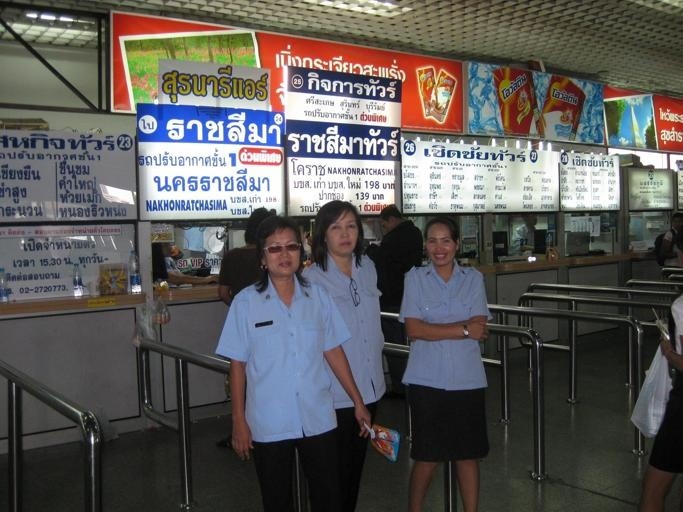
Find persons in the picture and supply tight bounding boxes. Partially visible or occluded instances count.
[161,243,218,285]
[301,200,387,511]
[367,205,424,400]
[396,216,494,511]
[639,294,682,511]
[510,213,537,256]
[215,216,372,511]
[216,208,271,448]
[662,213,682,293]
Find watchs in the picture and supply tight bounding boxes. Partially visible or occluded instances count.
[463,324,469,338]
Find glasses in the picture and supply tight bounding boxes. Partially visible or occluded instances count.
[261,242,304,253]
[347,276,361,308]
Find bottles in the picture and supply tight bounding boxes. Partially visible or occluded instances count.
[0,267,10,304]
[72,263,83,298]
[532,104,546,138]
[130,260,142,292]
